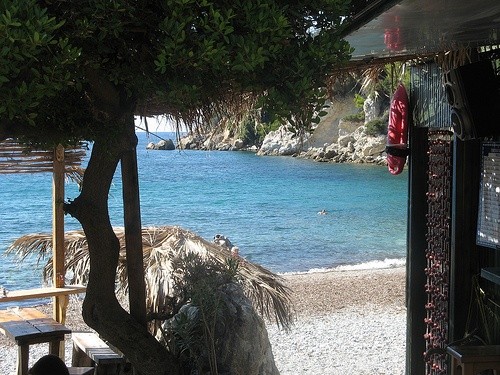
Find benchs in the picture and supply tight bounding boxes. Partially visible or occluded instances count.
[1,307,72,375]
[71,332,127,375]
[1,285,87,362]
[67,367,94,375]
[446,344,500,375]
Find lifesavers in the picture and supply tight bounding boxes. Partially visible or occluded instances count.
[384,28,404,50]
[387,83,408,175]
